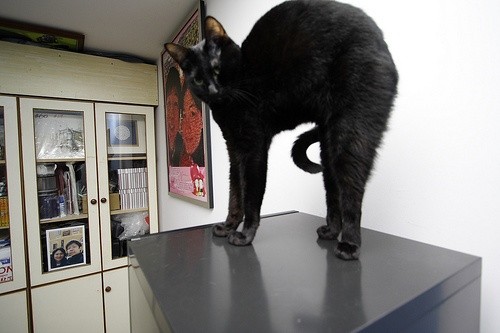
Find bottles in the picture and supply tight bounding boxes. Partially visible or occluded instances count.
[59,194,67,217]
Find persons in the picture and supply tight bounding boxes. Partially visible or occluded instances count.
[51,240,83,268]
[165,66,206,167]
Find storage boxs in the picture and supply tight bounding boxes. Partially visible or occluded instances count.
[83,194,120,214]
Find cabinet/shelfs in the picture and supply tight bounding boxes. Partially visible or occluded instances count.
[0,41,158,333]
[126,211,482,333]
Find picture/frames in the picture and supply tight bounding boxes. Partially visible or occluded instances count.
[160,0,214,210]
[105,113,145,154]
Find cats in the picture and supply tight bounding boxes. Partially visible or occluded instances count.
[165,0,399,257]
